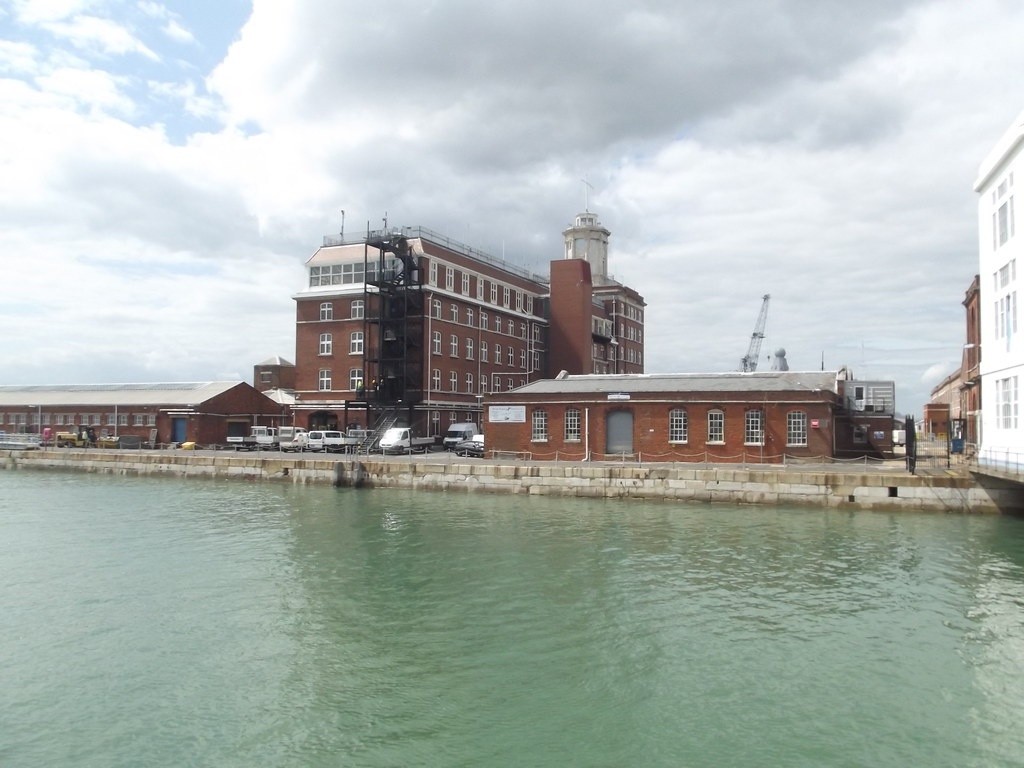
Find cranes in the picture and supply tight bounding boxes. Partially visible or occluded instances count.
[741,294,770,376]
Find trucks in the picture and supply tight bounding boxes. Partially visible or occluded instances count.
[891,430,906,447]
[227,426,277,451]
[379,427,435,455]
[444,422,478,449]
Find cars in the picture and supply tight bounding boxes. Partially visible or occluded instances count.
[455,435,484,457]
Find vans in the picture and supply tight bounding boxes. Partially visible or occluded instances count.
[279,426,307,449]
[308,431,346,452]
